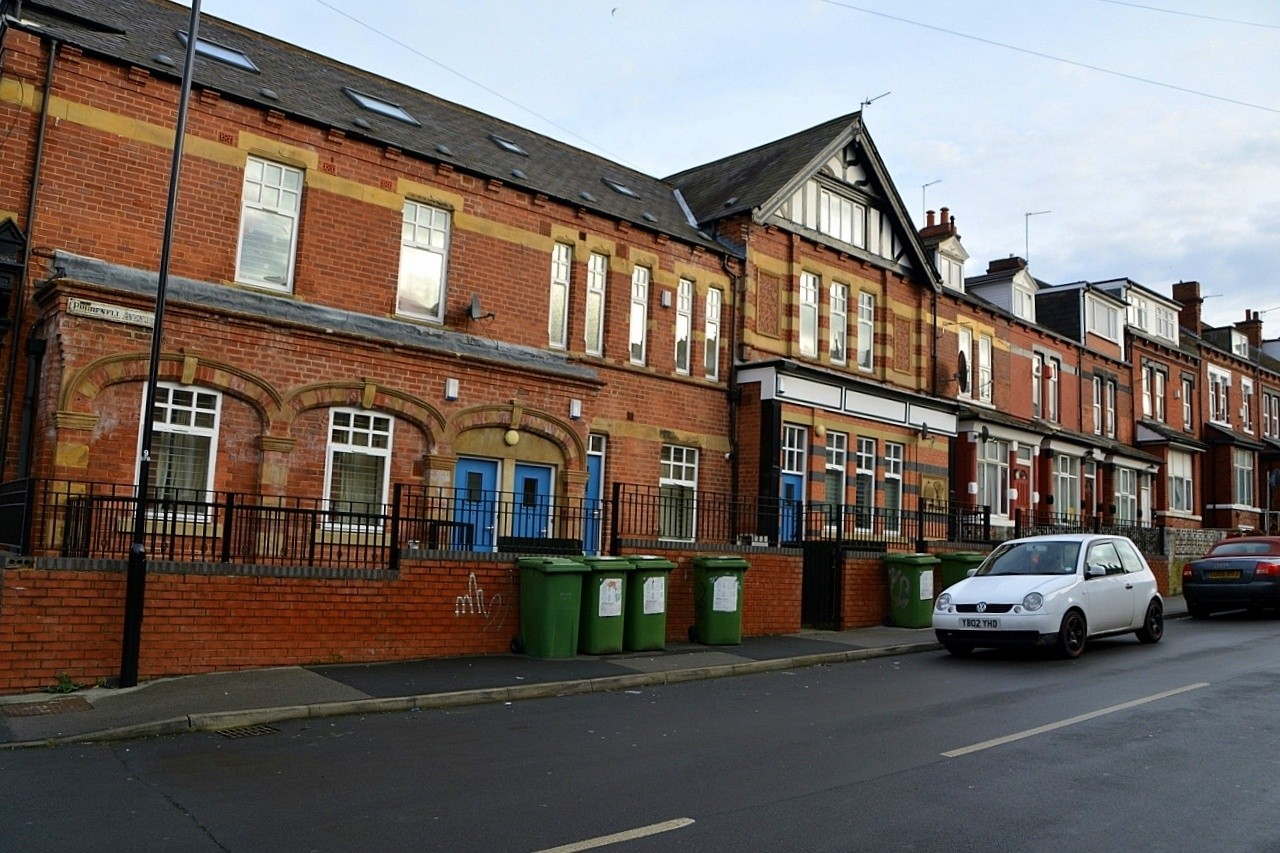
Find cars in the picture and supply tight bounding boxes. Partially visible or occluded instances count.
[933,535,1169,658]
[1181,537,1280,621]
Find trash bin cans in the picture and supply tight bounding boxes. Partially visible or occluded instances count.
[884,554,942,629]
[623,556,678,651]
[510,558,591,658]
[570,556,635,655]
[937,552,986,591]
[688,556,750,643]
